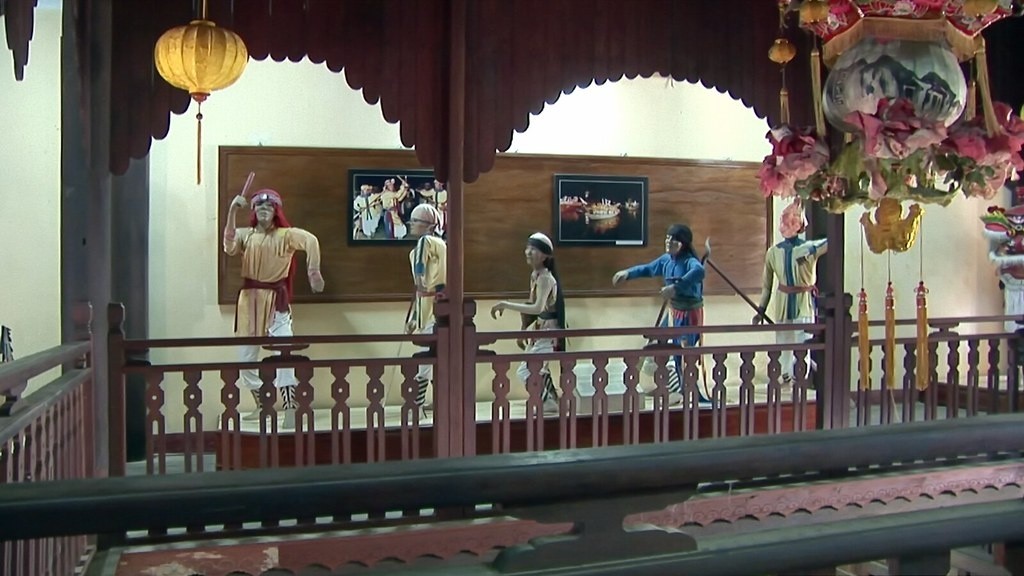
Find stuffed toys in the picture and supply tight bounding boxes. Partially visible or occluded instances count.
[979,204,1024,333]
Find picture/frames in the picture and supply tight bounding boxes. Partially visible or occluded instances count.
[552,174,648,248]
[347,168,448,245]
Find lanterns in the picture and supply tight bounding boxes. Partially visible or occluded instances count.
[753,0,1024,392]
[154,0,247,186]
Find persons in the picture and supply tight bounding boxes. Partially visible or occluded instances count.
[750,201,827,389]
[405,204,447,423]
[223,189,325,428]
[353,176,448,239]
[490,232,565,414]
[613,225,715,405]
